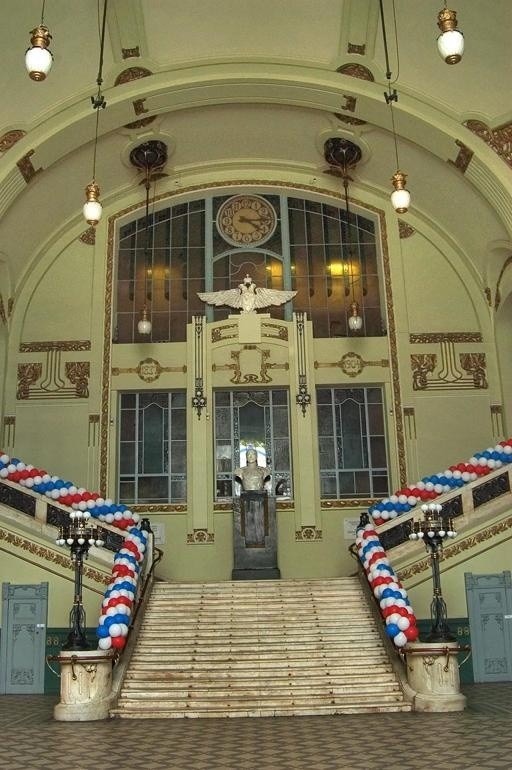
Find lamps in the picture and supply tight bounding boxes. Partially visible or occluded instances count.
[322,136,363,331]
[379,0,411,213]
[436,0,465,65]
[25,0,55,82]
[82,0,108,227]
[130,140,170,336]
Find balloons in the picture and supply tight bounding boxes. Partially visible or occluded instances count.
[354,439,512,647]
[0,453,149,650]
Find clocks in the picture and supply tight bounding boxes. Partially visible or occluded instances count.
[216,192,278,248]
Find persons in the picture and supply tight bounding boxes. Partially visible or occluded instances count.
[233,448,271,492]
